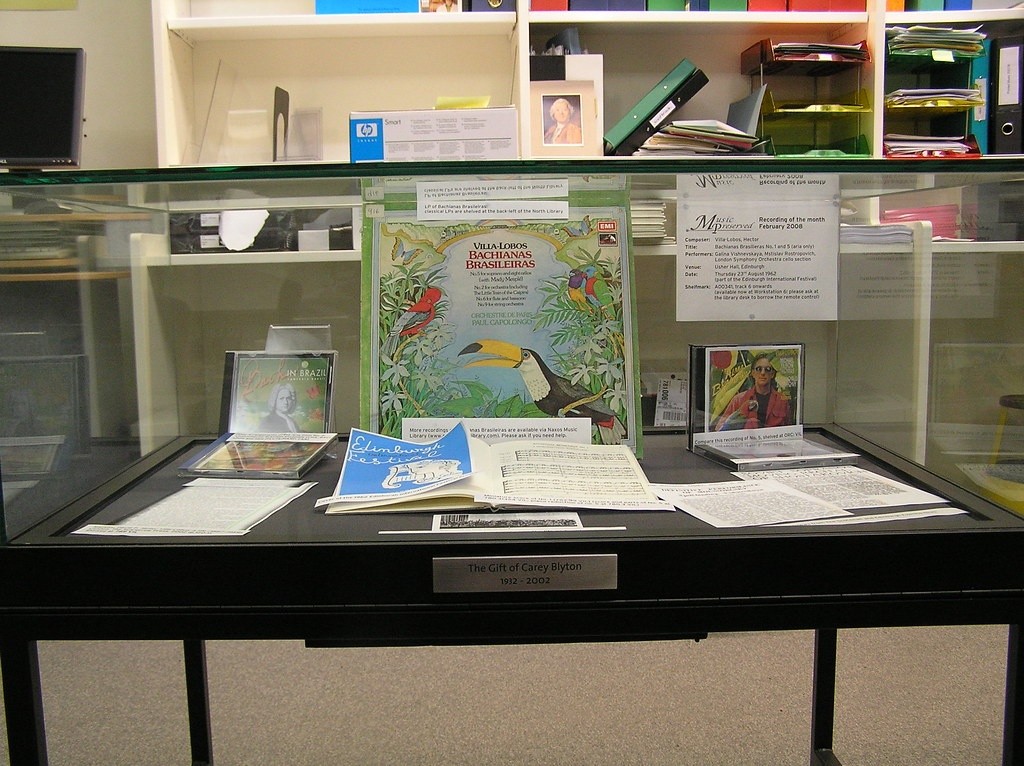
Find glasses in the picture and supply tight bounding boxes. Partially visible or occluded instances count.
[756,367,771,371]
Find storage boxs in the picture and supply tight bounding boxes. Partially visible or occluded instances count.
[350,108,518,163]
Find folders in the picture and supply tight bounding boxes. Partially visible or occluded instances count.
[604,58,709,156]
[546,26,581,53]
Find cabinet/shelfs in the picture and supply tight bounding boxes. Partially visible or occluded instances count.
[128,0,1024,467]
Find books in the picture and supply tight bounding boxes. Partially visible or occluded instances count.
[324,416,658,514]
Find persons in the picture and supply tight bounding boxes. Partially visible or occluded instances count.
[714,352,790,432]
[436,0,458,13]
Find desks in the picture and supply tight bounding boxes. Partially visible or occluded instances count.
[0,211,151,439]
[0,421,1024,766]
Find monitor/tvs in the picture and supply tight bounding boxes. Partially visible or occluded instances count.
[0,45,86,171]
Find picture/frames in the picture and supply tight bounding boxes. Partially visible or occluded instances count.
[218,350,338,461]
[686,343,806,452]
[529,79,598,156]
